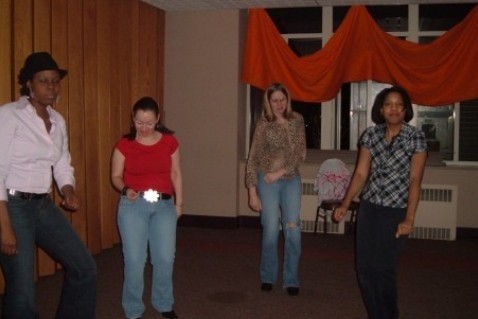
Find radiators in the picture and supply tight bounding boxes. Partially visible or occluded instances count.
[407,184,459,241]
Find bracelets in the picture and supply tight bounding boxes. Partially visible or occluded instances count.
[122,186,129,196]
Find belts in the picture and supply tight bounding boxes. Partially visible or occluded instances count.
[6,189,47,199]
[138,189,172,203]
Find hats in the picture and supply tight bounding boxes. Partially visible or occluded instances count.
[20,51,68,82]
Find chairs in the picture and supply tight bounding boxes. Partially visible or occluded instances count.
[313,158,360,246]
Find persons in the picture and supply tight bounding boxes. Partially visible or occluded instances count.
[110,96,182,319]
[243,82,309,297]
[0,51,99,319]
[333,86,427,319]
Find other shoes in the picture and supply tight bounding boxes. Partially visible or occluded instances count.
[162,310,179,319]
[261,283,272,291]
[287,286,300,296]
